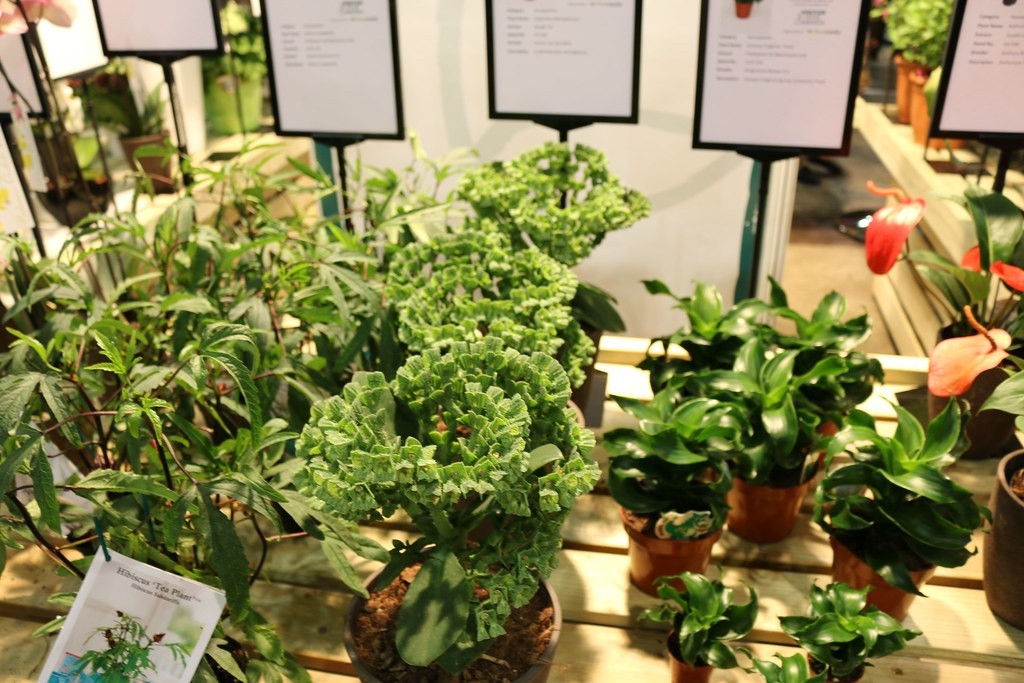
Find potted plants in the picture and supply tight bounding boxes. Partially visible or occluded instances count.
[0,0,1024,683]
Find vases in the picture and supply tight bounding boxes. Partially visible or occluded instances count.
[929,325,1024,458]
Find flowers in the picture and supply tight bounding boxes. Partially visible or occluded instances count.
[861,179,1024,397]
